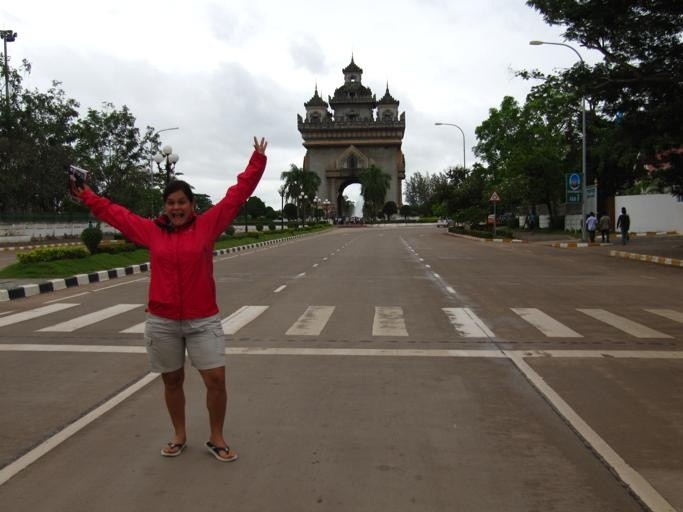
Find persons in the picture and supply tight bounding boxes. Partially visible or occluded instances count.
[585,212,598,243]
[67,136,267,462]
[598,211,612,243]
[616,207,631,245]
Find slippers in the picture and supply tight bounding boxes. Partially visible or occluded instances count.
[203,439,238,462]
[160,440,188,457]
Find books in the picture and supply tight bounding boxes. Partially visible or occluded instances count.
[66,164,89,207]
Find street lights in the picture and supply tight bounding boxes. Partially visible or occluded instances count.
[323,198,331,223]
[435,122,465,179]
[529,40,588,244]
[298,191,309,229]
[312,195,321,224]
[149,126,179,220]
